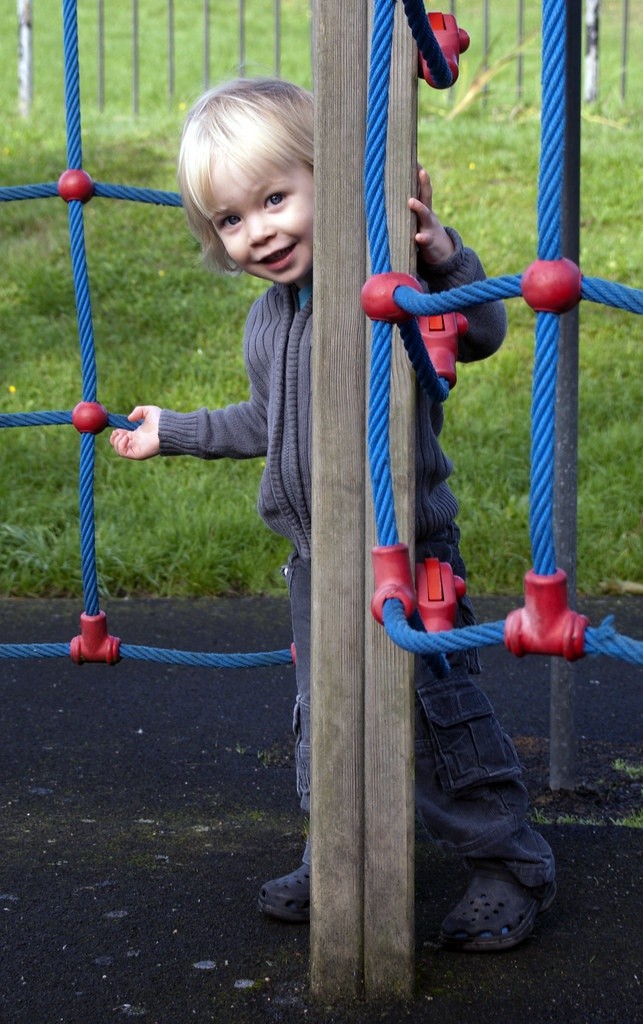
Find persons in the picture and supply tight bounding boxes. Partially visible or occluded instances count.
[109,74,559,952]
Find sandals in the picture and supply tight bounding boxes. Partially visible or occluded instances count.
[257,863,311,922]
[440,868,557,951]
